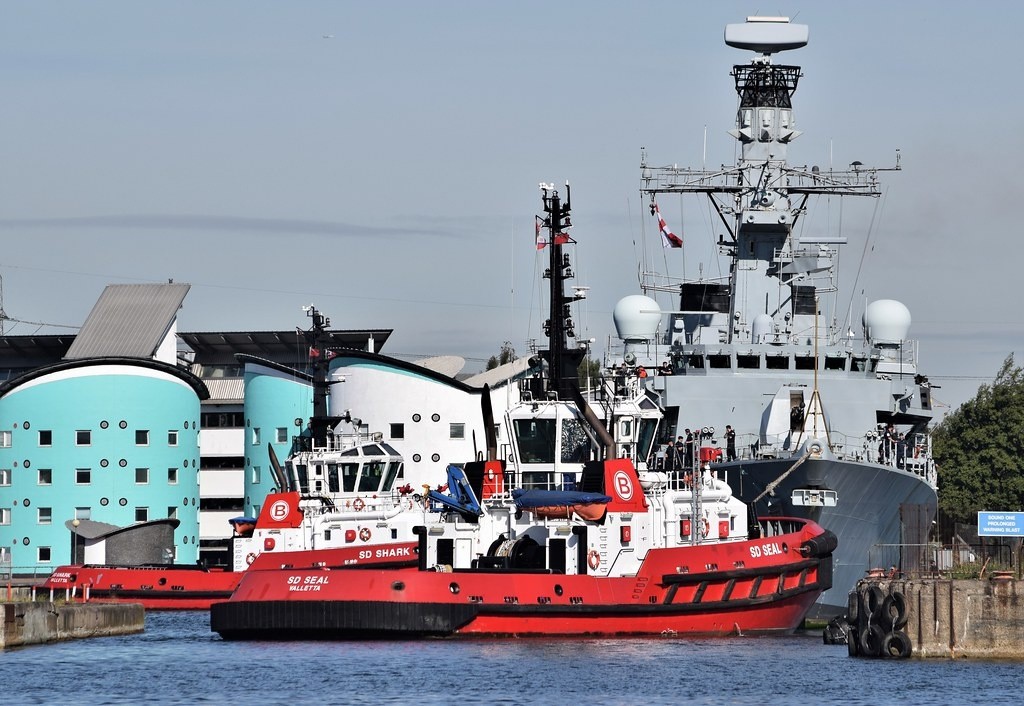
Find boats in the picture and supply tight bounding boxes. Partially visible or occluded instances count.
[208,175,840,645]
[511,490,613,520]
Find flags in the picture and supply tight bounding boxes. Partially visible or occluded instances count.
[310,348,319,356]
[537,237,546,250]
[328,351,336,359]
[656,203,683,248]
[555,234,569,244]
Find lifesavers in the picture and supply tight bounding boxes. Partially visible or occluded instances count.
[848,592,859,621]
[823,629,832,644]
[847,629,858,656]
[246,553,257,565]
[588,550,600,569]
[702,518,709,537]
[863,585,885,620]
[360,528,371,540]
[860,624,886,656]
[881,630,912,658]
[882,591,910,628]
[409,500,412,510]
[353,499,364,510]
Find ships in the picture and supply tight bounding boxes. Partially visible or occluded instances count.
[613,15,941,630]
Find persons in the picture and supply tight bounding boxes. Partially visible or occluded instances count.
[724,425,736,462]
[897,432,907,468]
[685,429,693,466]
[888,565,898,577]
[659,362,672,376]
[665,440,674,469]
[675,436,683,466]
[884,424,898,458]
[638,365,648,378]
